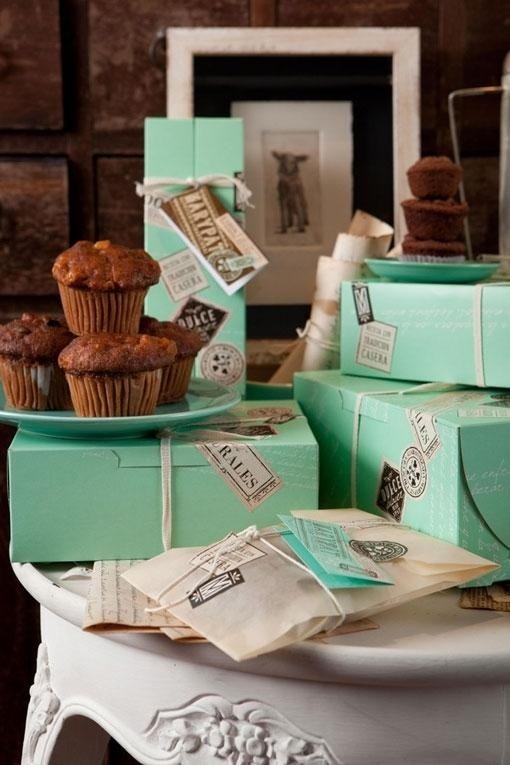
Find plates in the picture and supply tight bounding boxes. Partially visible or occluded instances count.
[365,257,499,282]
[0,376,241,440]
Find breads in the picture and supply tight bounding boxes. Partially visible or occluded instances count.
[399,154,469,255]
[1,238,203,417]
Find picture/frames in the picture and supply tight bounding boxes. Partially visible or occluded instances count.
[161,23,421,370]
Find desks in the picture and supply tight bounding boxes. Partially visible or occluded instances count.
[4,534,510,764]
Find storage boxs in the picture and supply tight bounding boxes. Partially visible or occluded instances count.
[5,398,323,564]
[296,371,507,586]
[134,111,255,400]
[331,279,510,394]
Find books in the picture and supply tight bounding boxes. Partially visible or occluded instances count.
[159,182,271,295]
